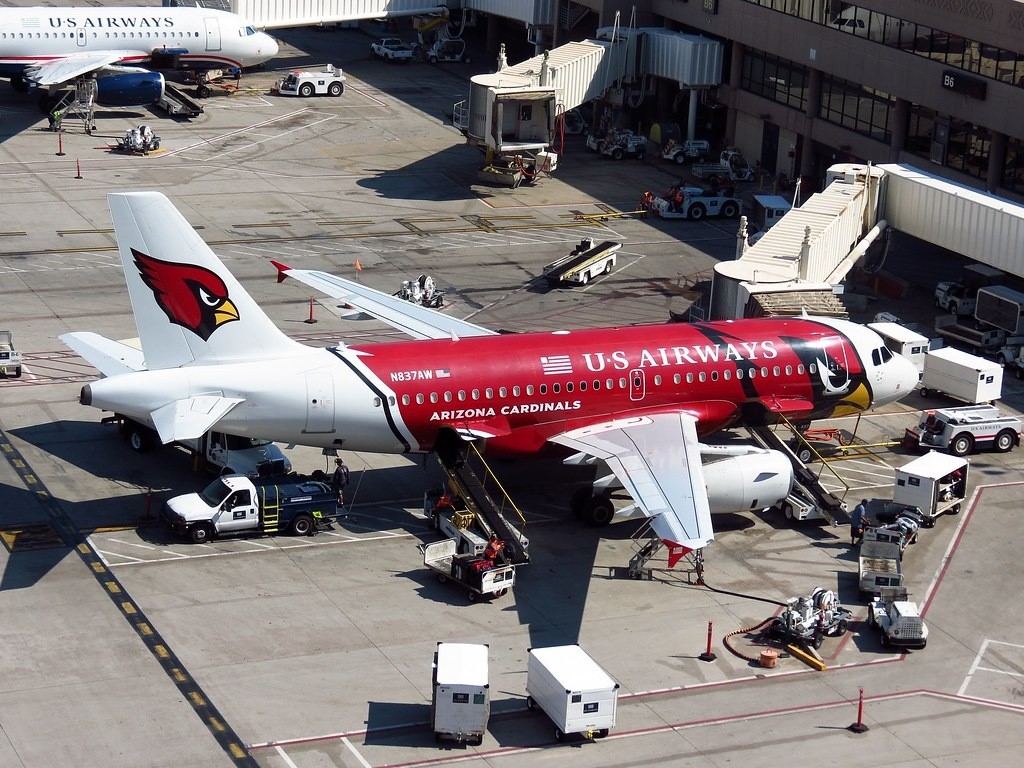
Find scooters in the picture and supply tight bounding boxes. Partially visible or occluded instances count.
[691,151,756,184]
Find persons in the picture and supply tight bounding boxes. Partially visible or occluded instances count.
[131,124,156,156]
[413,43,424,63]
[710,176,736,197]
[640,182,684,219]
[333,458,351,508]
[851,498,871,546]
[485,536,504,567]
[778,172,810,193]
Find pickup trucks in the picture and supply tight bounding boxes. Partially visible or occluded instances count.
[160,473,350,545]
[97,407,293,478]
[370,37,414,63]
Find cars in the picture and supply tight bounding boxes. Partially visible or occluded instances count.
[1,331,22,379]
[584,128,648,160]
[660,140,710,164]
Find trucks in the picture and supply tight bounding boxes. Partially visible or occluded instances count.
[422,488,532,559]
[223,68,242,79]
[862,316,1023,648]
[417,537,518,601]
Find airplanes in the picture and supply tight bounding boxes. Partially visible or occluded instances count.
[57,193,918,567]
[1,5,279,97]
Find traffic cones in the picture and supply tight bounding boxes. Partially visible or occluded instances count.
[54,133,66,156]
[74,158,84,179]
